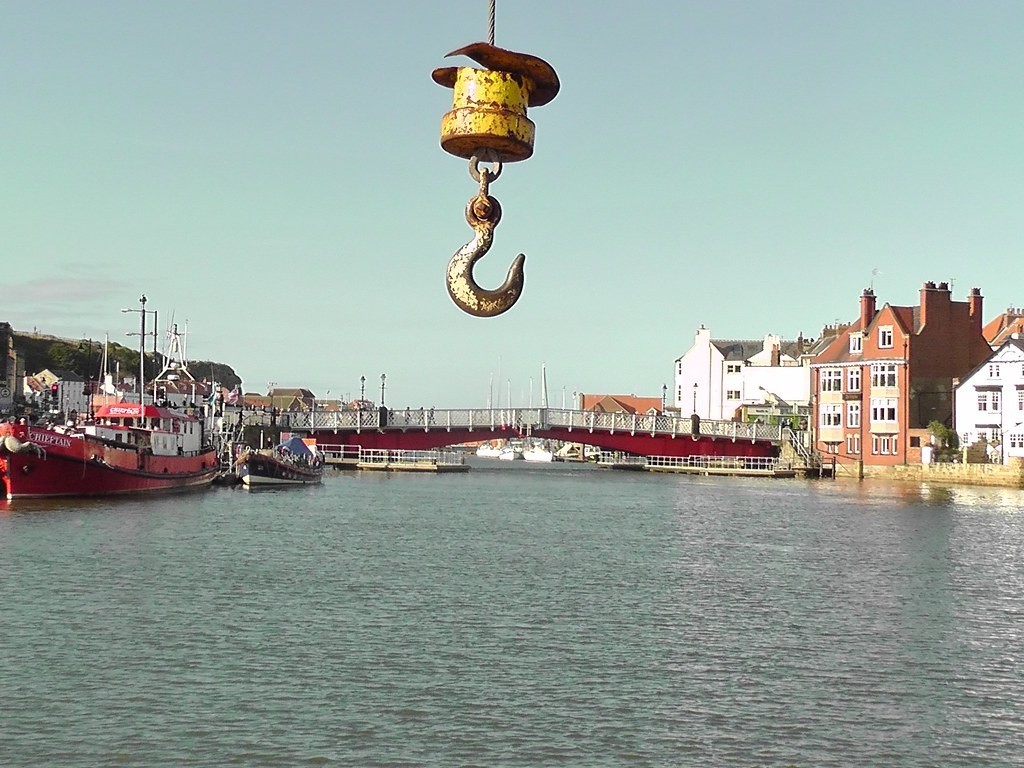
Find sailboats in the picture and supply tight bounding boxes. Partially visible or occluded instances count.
[475,365,560,462]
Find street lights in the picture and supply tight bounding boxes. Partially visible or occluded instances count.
[360,375,366,402]
[380,373,388,406]
[661,384,667,417]
[89,372,95,420]
[693,382,698,415]
[78,337,103,421]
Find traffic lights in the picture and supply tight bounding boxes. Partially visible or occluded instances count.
[52,384,58,397]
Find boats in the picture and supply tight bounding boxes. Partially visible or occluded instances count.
[238,437,322,486]
[0,293,223,501]
